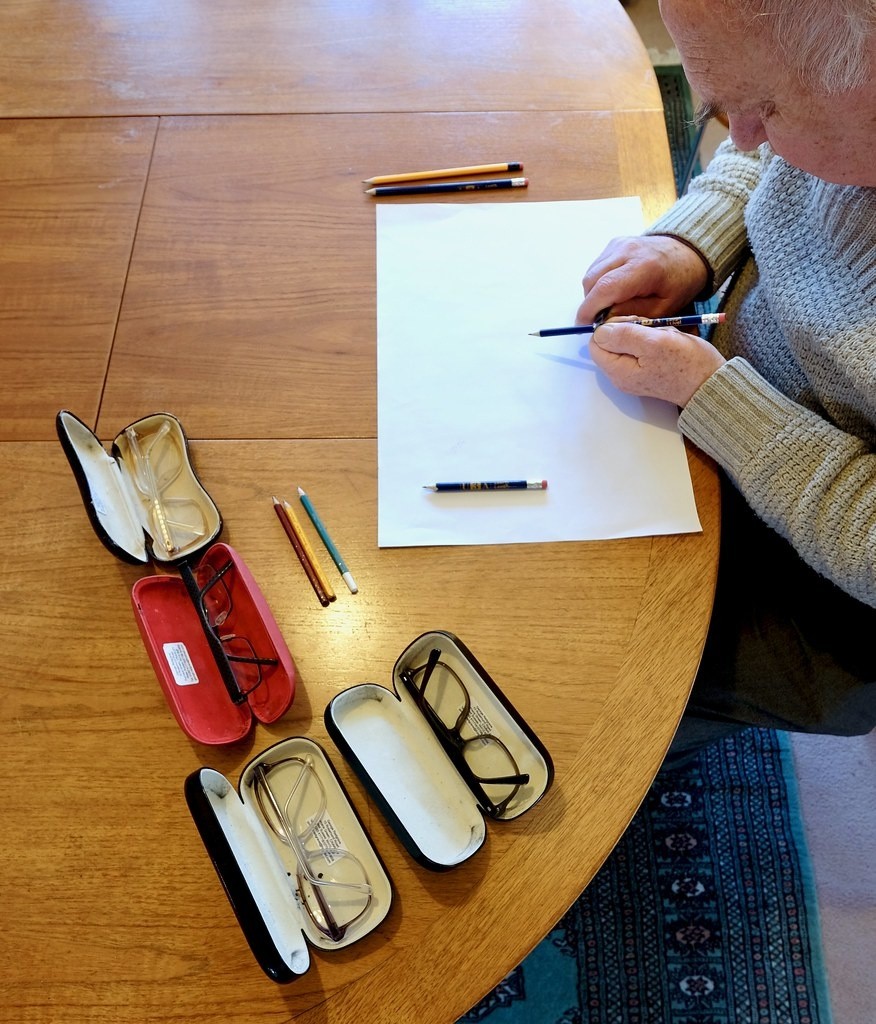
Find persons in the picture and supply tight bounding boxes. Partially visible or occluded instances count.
[576,0,876,738]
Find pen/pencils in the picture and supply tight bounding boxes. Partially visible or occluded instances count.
[528,311,725,338]
[296,486,358,594]
[363,177,529,196]
[423,479,548,492]
[361,160,523,184]
[281,499,336,602]
[272,496,330,606]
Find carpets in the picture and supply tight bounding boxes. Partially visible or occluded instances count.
[455,65,831,1024]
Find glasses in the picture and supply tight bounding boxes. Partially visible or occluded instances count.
[249,758,372,941]
[180,561,278,705]
[125,421,207,554]
[399,649,530,817]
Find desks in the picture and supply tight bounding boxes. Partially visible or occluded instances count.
[0,0,720,1024]
